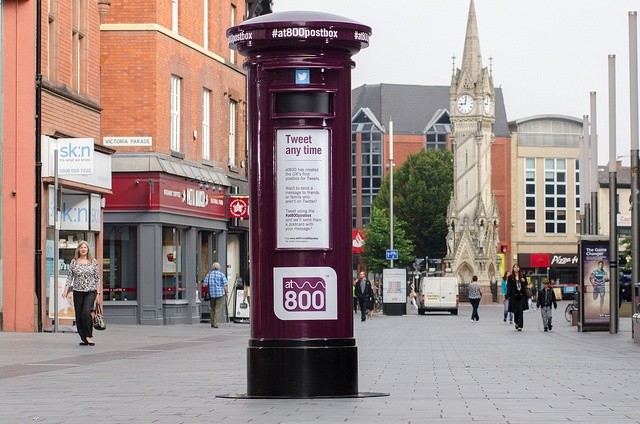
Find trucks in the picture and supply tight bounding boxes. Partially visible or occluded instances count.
[416,276,459,315]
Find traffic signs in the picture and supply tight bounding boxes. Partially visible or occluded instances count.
[386,249,398,260]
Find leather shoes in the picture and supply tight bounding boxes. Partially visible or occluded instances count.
[515,324,518,329]
[518,328,522,331]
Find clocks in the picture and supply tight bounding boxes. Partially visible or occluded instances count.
[483,94,492,115]
[456,93,475,115]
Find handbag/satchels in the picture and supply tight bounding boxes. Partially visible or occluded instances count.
[204,272,211,301]
[409,288,417,298]
[90,302,106,330]
[521,274,532,298]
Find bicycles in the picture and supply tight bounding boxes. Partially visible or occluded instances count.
[564,299,578,322]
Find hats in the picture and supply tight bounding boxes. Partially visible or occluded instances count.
[543,278,550,284]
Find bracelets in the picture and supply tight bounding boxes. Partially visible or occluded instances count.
[96,291,100,295]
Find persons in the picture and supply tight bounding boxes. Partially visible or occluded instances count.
[502,263,528,331]
[590,258,609,317]
[62,240,104,347]
[355,270,373,321]
[468,274,483,322]
[501,270,514,325]
[203,261,229,328]
[58,285,76,316]
[355,277,359,282]
[535,278,557,332]
[407,279,420,314]
[353,279,358,313]
[241,267,252,314]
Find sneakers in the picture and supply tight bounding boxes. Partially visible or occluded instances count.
[549,325,552,330]
[544,327,548,332]
